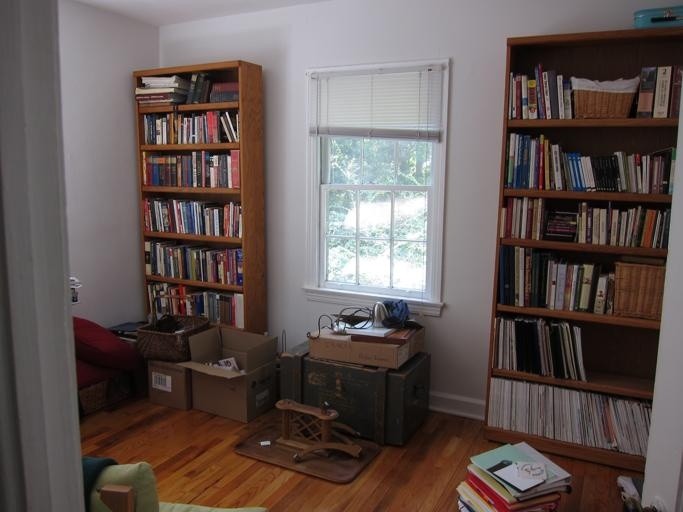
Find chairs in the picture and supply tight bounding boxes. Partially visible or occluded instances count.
[81,456,268,512]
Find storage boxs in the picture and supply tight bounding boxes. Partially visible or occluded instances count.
[175,327,278,423]
[279,339,432,448]
[117,336,149,398]
[309,314,426,370]
[147,360,194,411]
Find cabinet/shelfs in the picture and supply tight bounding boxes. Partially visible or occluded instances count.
[133,61,269,333]
[481,26,683,475]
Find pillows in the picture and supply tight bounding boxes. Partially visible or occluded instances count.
[72,316,140,388]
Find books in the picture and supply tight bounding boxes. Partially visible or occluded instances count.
[134,72,244,331]
[453,440,572,512]
[348,325,418,344]
[637,63,679,119]
[505,132,677,196]
[499,246,665,320]
[508,63,573,120]
[499,197,671,250]
[488,378,651,456]
[491,316,588,382]
[345,320,395,338]
[217,356,238,370]
